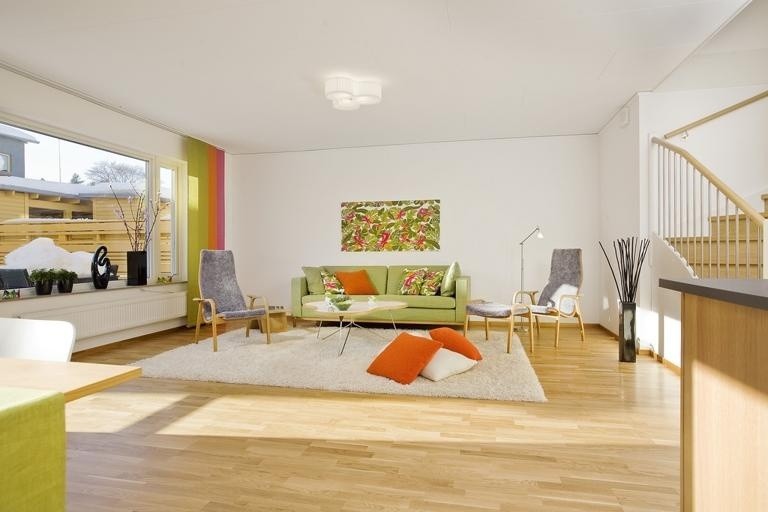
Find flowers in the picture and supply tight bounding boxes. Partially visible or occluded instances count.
[108,184,172,252]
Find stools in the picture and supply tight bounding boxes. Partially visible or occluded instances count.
[463,298,535,353]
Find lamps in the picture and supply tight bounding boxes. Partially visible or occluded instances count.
[514,227,544,333]
[325,74,383,112]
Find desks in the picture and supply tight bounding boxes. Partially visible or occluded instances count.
[0,355,144,512]
[659,279,768,512]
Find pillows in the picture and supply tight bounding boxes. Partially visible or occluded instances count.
[366,332,443,385]
[440,262,462,297]
[332,269,380,296]
[302,266,326,295]
[419,346,478,381]
[419,269,443,296]
[429,328,483,363]
[396,269,426,295]
[320,271,345,296]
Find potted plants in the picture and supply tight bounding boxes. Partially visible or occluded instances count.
[30,269,54,296]
[55,270,79,293]
[598,236,654,363]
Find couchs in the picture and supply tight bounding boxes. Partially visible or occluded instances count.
[290,262,472,330]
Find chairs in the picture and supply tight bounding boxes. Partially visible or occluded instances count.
[0,317,76,361]
[512,249,586,348]
[192,248,271,353]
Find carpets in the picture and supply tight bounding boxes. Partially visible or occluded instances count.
[121,326,548,403]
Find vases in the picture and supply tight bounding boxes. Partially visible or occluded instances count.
[127,250,149,286]
